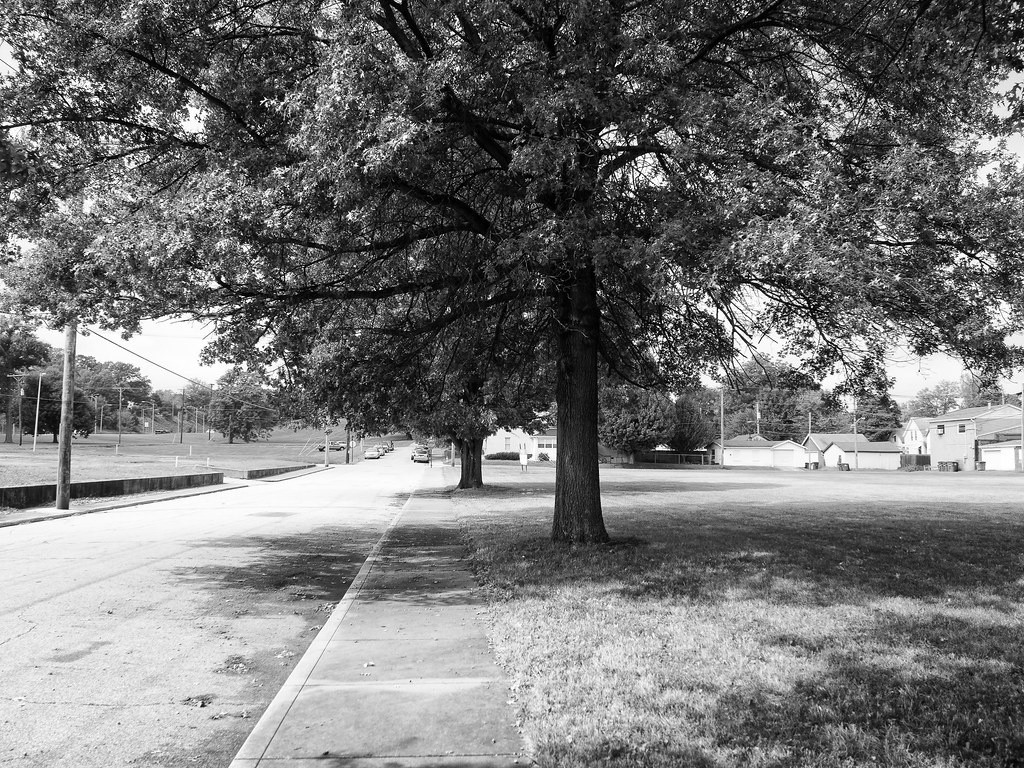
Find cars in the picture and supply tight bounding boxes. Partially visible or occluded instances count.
[410,442,430,463]
[363,439,394,459]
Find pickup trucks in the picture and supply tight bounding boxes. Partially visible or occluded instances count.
[318,440,348,452]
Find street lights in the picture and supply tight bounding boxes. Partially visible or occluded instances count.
[187,406,197,433]
[32,372,46,453]
[141,400,155,435]
[84,395,97,435]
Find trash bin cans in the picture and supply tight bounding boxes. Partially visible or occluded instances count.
[843,463,849,471]
[938,461,958,472]
[805,462,810,469]
[976,461,986,471]
[812,462,819,469]
